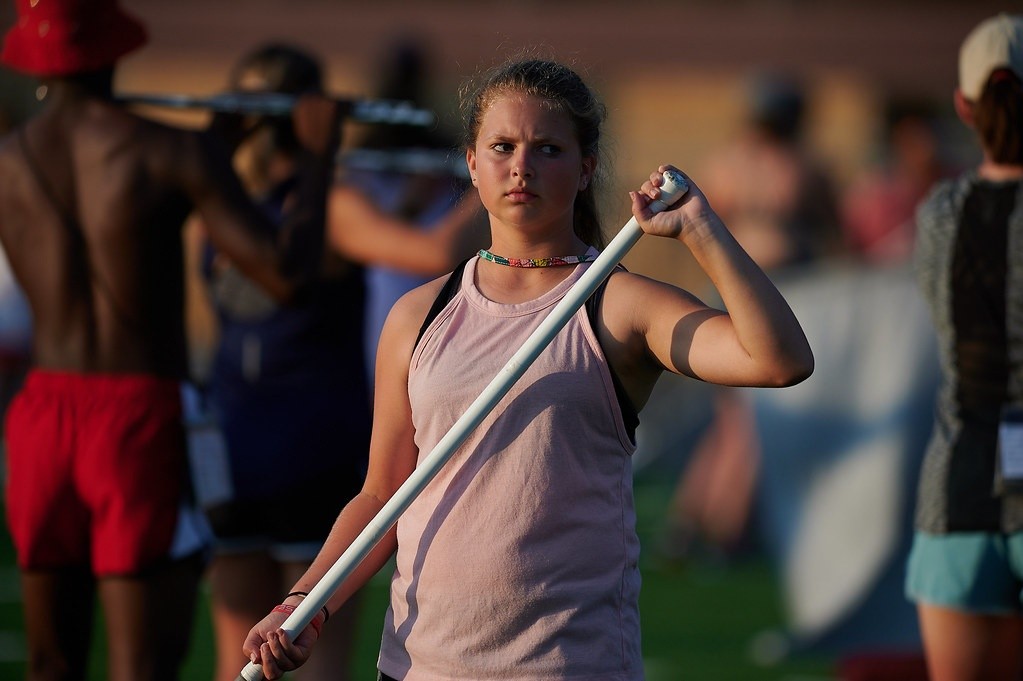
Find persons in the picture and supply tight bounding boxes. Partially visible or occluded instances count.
[242,47,817,681]
[0,0,1023,681]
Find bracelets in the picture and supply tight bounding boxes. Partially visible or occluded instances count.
[284,591,330,624]
[271,605,322,640]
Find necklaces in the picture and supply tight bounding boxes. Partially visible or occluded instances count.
[477,247,631,273]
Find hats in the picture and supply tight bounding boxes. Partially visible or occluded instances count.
[958,10,1023,102]
[1,0,150,79]
